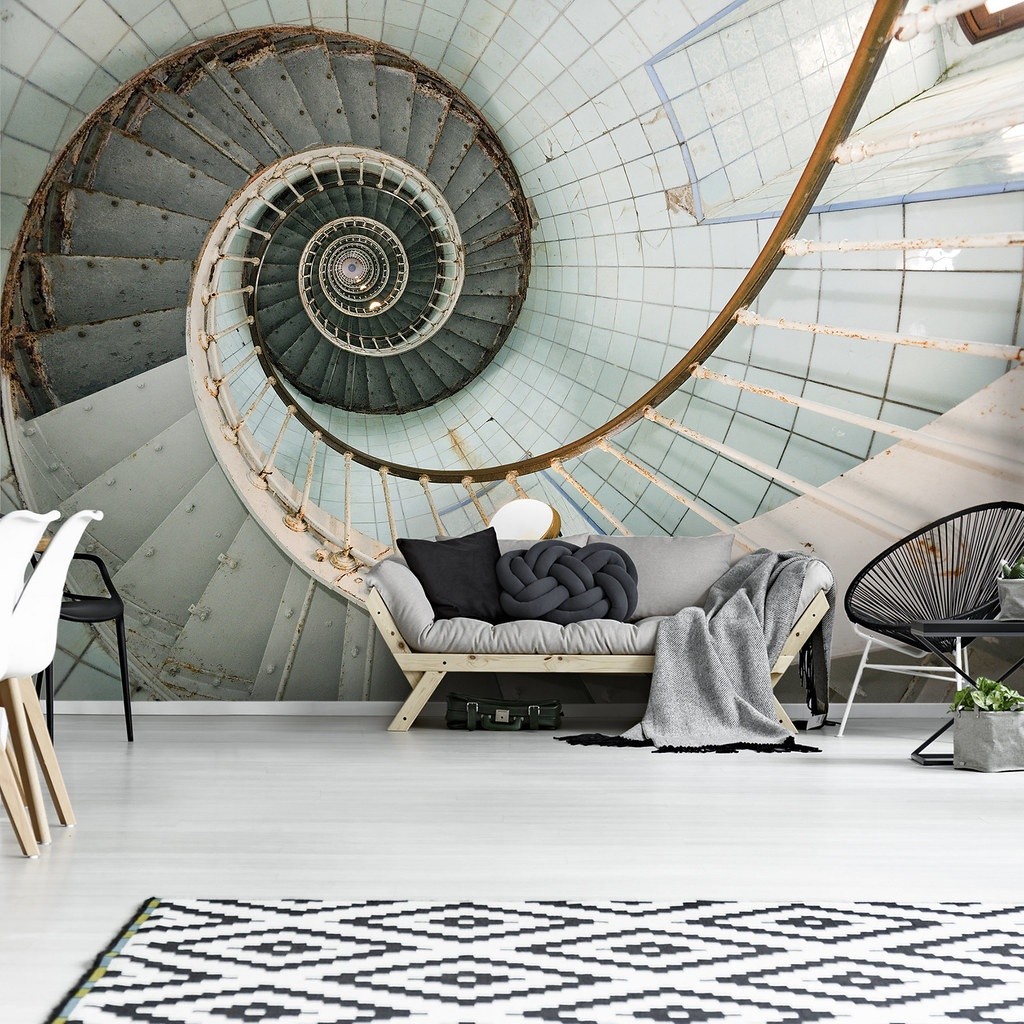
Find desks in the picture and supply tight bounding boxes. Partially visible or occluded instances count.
[910,619,1024,767]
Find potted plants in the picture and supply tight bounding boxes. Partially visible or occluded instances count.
[993,556,1024,621]
[946,676,1024,773]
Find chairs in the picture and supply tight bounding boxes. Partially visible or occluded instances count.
[834,500,1024,739]
[6,509,104,826]
[0,509,61,859]
[30,537,134,746]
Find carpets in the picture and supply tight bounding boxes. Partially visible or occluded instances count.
[42,895,1024,1024]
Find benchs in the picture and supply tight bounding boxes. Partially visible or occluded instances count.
[364,543,833,734]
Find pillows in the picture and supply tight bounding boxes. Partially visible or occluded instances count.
[396,526,502,625]
[435,533,590,555]
[588,533,737,622]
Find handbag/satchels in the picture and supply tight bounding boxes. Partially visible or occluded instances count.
[446,692,564,731]
[994,560,1024,621]
[954,702,1024,773]
